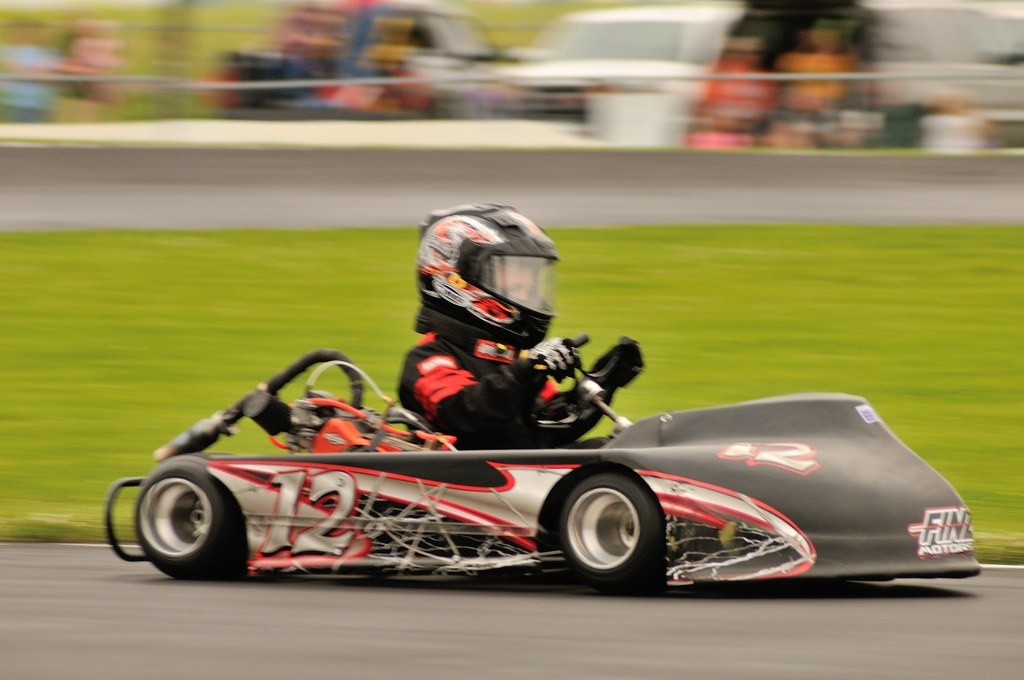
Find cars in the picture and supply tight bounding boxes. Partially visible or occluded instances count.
[519,8,750,152]
[192,4,589,124]
[861,2,1023,148]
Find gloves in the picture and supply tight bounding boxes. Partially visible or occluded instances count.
[584,340,643,390]
[526,334,583,384]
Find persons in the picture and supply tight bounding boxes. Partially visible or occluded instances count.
[397,201,642,449]
[1,19,127,124]
[682,20,863,152]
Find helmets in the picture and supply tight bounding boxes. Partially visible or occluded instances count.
[412,201,559,351]
